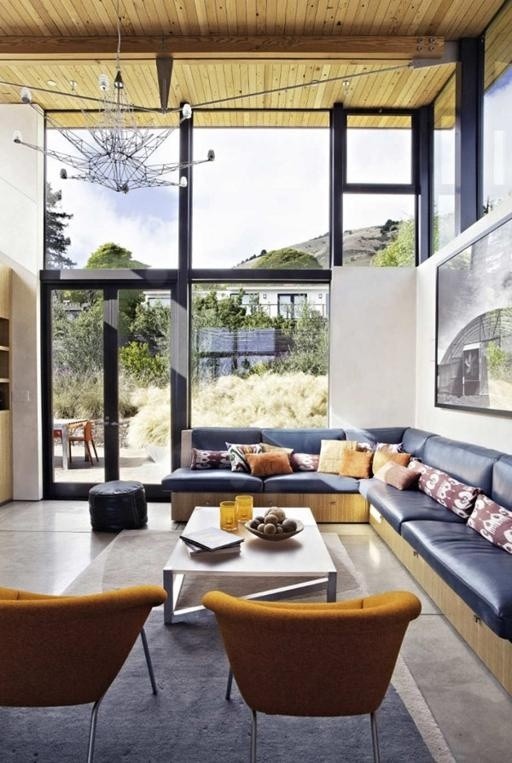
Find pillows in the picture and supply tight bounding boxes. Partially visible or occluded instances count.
[191,439,421,490]
[465,494,512,556]
[406,460,482,518]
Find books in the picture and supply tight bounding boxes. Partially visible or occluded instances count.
[179,527,245,558]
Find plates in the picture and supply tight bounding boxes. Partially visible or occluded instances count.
[244,519,304,541]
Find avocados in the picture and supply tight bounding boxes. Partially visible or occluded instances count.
[250,509,297,535]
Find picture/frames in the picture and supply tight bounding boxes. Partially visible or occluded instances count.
[435,212,511,420]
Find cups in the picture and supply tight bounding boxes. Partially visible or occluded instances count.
[234,496,253,524]
[220,500,237,532]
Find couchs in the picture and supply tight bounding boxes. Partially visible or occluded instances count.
[162,425,512,700]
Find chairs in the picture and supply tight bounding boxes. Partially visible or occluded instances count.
[200,588,423,762]
[69,421,100,467]
[0,580,171,762]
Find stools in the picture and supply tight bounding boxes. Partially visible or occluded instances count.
[88,479,149,532]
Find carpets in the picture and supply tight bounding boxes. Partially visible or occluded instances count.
[1,527,457,763]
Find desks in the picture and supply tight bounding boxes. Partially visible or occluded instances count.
[53,418,86,470]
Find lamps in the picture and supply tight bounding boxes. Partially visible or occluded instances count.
[12,53,217,197]
